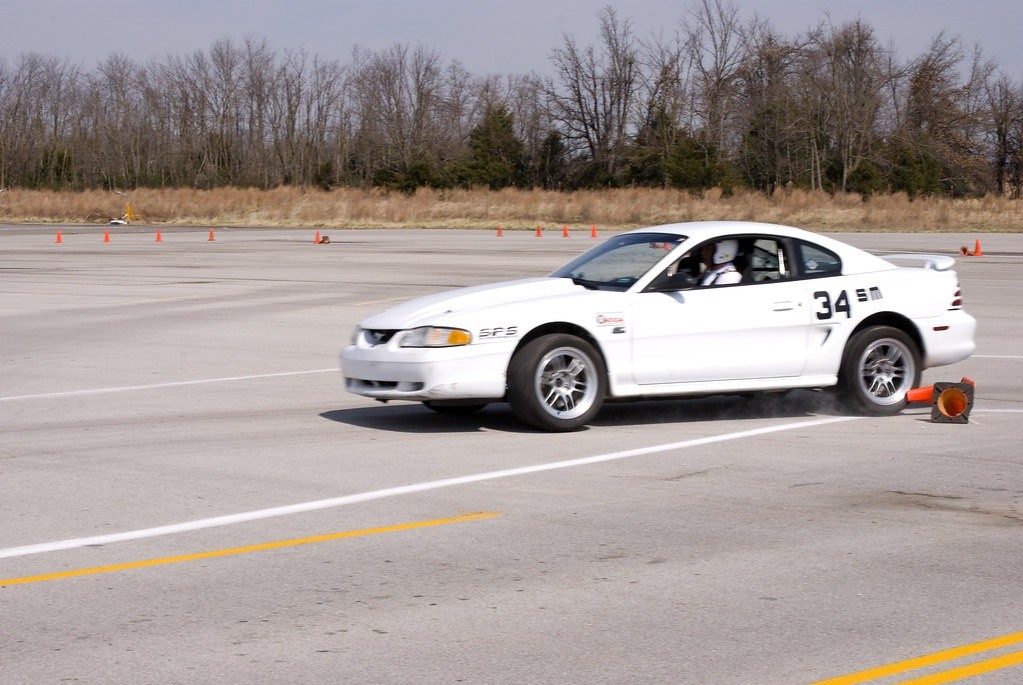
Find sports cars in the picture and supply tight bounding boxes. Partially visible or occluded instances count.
[338,219,979,432]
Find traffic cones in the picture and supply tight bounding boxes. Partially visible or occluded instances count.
[153,228,163,242]
[591,226,597,238]
[534,224,543,238]
[496,224,505,237]
[104,229,111,243]
[55,230,64,243]
[959,246,975,257]
[562,226,570,238]
[929,381,975,425]
[320,235,331,244]
[973,239,983,258]
[905,377,977,405]
[661,242,669,250]
[651,241,662,249]
[314,230,322,245]
[206,228,215,241]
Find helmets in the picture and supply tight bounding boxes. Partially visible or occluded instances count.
[712,239,738,265]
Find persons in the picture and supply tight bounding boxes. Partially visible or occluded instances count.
[699,239,743,286]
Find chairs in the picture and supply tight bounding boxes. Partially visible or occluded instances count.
[677,257,701,277]
